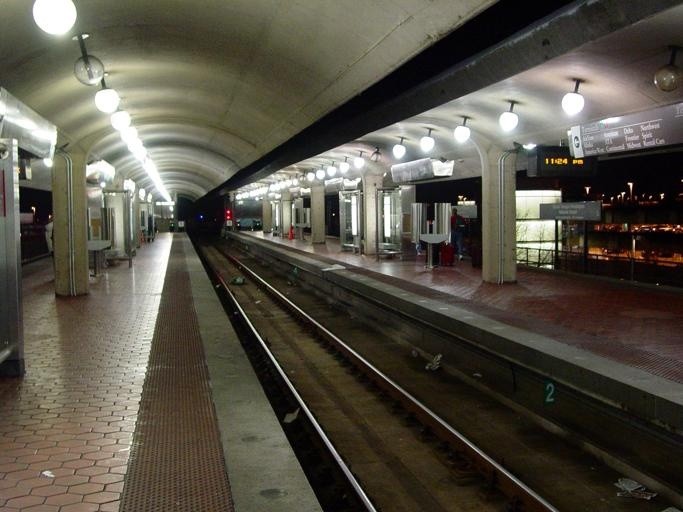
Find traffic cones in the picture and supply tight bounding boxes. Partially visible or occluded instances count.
[289,225,293,240]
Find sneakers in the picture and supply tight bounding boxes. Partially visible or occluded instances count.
[459,255,463,261]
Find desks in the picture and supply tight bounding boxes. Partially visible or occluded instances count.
[86,238,112,276]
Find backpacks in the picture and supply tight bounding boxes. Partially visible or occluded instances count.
[455,215,464,232]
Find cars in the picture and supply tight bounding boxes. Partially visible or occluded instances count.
[605,223,683,233]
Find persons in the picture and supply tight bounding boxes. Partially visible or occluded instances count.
[45,213,54,270]
[451,207,467,261]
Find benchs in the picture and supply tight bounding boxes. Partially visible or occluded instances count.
[343,244,359,255]
[377,251,404,262]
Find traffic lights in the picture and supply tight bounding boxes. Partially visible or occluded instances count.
[226,210,231,220]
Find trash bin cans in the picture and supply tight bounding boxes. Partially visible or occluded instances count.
[169,223,177,232]
[441,243,455,265]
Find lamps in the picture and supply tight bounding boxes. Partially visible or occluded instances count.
[653,43,683,94]
[31,0,132,132]
[306,79,585,182]
